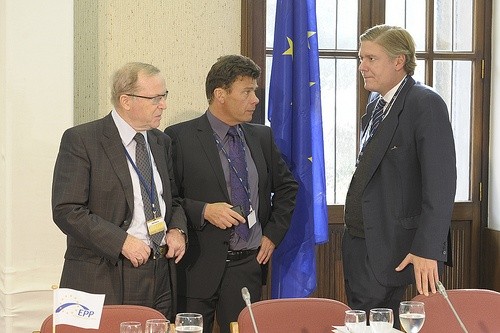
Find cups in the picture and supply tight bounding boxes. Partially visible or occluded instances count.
[120,321,141,333]
[175,313,204,333]
[345,310,367,333]
[399,301,425,333]
[145,319,172,333]
[369,308,395,333]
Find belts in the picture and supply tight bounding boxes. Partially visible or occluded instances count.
[225,248,258,262]
[150,245,169,260]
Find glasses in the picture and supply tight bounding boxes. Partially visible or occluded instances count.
[126,90,169,105]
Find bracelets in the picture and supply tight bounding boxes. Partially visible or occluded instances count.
[177,228,185,234]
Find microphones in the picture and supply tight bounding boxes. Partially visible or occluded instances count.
[436,281,469,333]
[242,287,258,333]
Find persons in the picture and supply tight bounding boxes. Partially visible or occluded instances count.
[342,24,457,330]
[164,55,299,333]
[52,62,188,324]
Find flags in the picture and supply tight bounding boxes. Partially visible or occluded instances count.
[268,0,329,299]
[53,288,106,330]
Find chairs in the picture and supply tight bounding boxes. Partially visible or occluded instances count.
[237,298,355,333]
[40,305,168,333]
[400,288,500,333]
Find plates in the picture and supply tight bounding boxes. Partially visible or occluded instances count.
[330,325,406,333]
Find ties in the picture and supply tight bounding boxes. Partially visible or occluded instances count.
[226,125,254,243]
[133,133,165,245]
[369,98,386,132]
